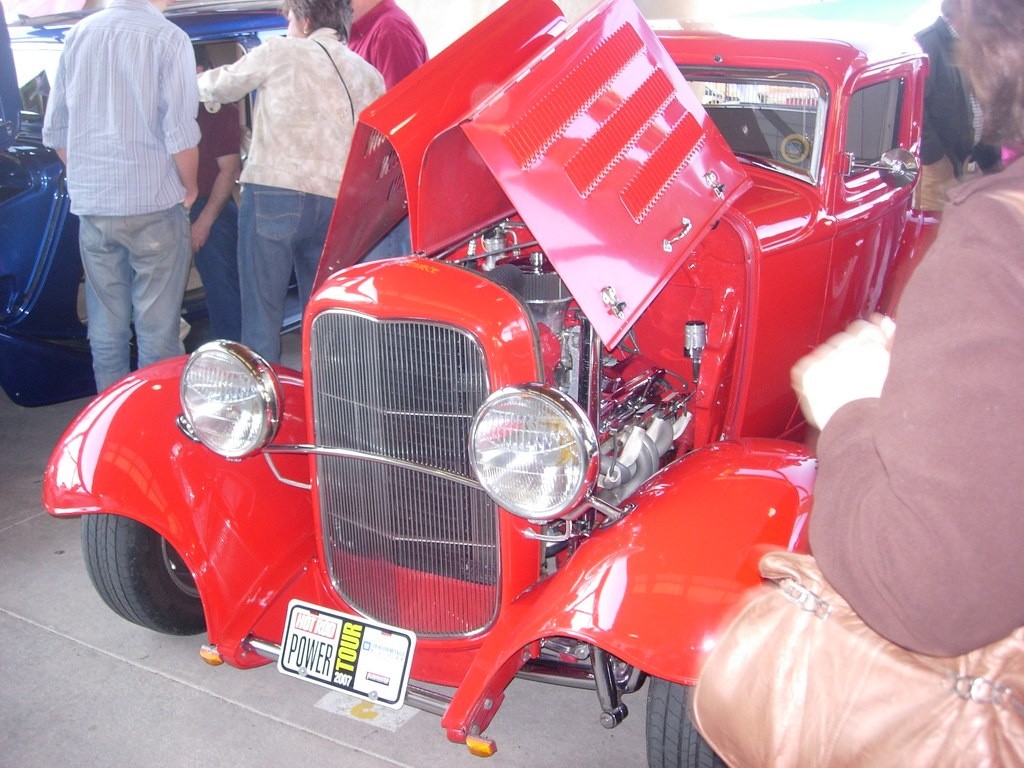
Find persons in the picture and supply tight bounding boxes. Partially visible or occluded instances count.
[792,0,1024,649]
[198,0,385,362]
[42,0,200,395]
[915,0,983,210]
[343,0,427,264]
[191,40,244,347]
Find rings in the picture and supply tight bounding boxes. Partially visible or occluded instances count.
[195,244,198,248]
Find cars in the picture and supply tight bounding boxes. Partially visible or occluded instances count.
[42,27,943,764]
[0,4,303,403]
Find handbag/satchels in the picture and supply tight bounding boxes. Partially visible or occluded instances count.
[689,547,1020,767]
